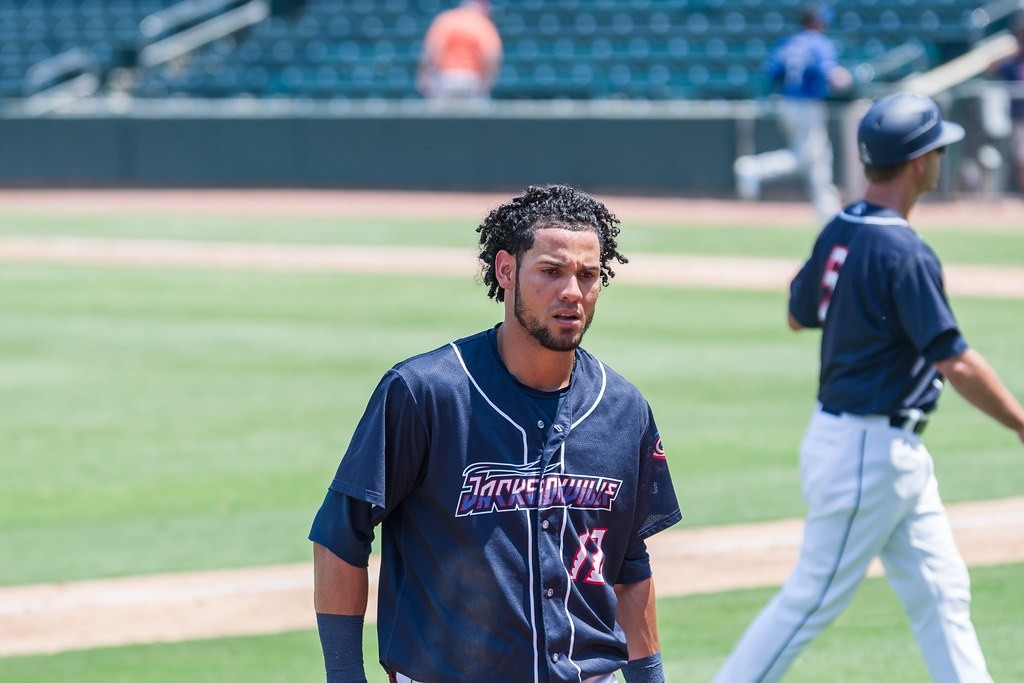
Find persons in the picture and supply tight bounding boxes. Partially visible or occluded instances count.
[308,183,682,683]
[416,0,502,100]
[716,90,1024,683]
[734,0,852,220]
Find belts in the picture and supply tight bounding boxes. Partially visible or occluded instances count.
[822,406,927,435]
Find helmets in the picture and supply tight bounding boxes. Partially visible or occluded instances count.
[858,92,965,167]
